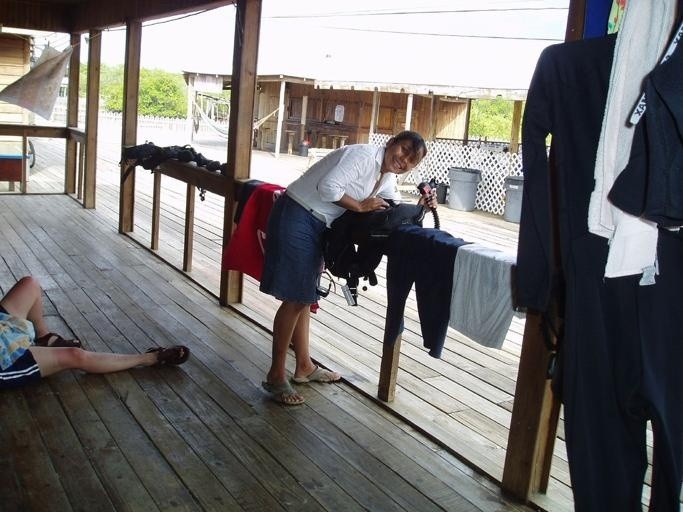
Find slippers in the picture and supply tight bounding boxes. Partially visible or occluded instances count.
[291,364,342,385]
[260,377,305,406]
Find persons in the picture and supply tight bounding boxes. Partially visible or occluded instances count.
[259,130,438,405]
[0,277,191,386]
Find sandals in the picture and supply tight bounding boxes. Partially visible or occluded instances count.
[34,333,81,348]
[146,346,189,369]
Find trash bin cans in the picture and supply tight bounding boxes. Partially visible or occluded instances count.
[298,141,311,157]
[435,183,450,204]
[503,176,524,224]
[447,167,482,212]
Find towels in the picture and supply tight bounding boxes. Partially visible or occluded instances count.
[0,43,73,121]
[587,0,682,287]
